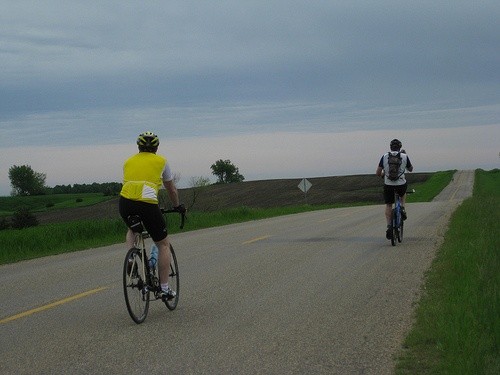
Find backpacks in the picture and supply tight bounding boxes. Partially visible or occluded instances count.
[387,152,405,181]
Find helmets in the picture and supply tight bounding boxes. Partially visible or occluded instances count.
[390,139,402,148]
[137,131,160,147]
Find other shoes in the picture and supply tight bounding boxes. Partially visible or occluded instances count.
[386,226,393,239]
[399,208,407,220]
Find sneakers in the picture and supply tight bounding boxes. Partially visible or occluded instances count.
[161,288,176,301]
[127,259,138,279]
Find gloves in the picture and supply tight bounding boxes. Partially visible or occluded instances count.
[174,203,185,213]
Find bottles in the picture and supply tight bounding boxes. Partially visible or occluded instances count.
[150,244,158,269]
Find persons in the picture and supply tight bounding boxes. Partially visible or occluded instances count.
[118,132,183,300]
[376,139,413,240]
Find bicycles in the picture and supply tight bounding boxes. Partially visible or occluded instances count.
[381,187,415,246]
[122,206,188,324]
